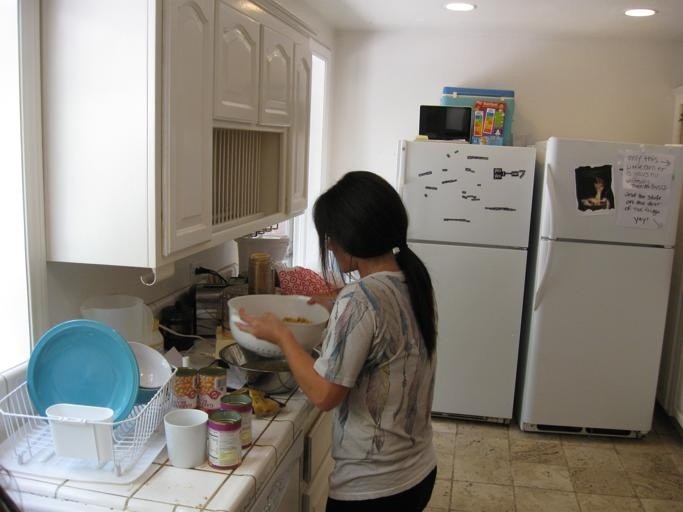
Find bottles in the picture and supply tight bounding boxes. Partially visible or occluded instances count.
[161,301,193,351]
[171,367,199,410]
[198,367,227,414]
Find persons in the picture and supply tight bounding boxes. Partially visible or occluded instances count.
[233,170,438,512]
[581,171,610,208]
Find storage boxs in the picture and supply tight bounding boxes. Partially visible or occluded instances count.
[441,87,515,145]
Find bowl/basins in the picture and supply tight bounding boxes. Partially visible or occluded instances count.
[219,342,321,393]
[227,294,330,357]
[128,342,172,388]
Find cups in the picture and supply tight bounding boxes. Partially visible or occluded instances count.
[164,409,209,468]
[249,253,276,294]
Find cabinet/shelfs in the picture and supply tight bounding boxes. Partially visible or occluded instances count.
[263,15,312,217]
[41,0,212,269]
[240,408,334,512]
[213,0,295,133]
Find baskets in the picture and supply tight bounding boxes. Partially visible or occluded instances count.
[0,363,178,478]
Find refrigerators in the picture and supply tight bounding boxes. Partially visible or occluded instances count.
[520,137,683,439]
[397,140,536,426]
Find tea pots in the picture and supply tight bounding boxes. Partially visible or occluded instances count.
[80,294,153,346]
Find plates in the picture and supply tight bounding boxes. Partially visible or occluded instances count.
[26,319,140,429]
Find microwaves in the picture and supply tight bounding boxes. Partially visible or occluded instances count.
[418,104,475,143]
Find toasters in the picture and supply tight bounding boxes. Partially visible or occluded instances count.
[188,283,227,336]
